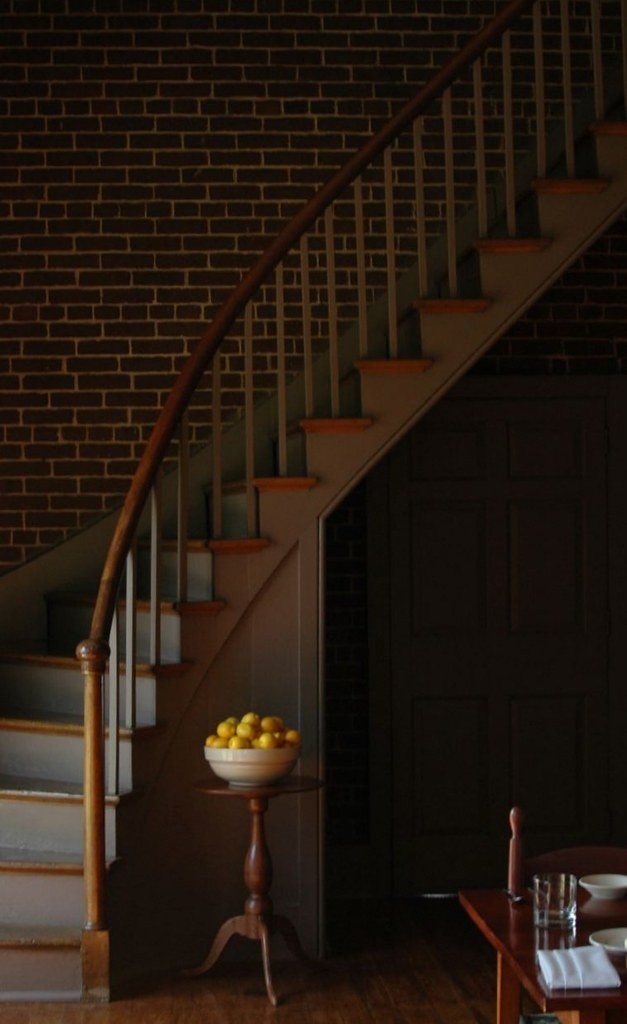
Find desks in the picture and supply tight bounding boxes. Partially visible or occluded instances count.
[178,777,323,1007]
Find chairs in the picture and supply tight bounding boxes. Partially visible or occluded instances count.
[455,805,627,1024]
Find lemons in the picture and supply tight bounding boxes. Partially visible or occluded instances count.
[206,712,300,749]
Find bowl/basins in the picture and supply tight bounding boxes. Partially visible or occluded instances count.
[579,873,627,899]
[204,746,300,787]
[580,898,627,917]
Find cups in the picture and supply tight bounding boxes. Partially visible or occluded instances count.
[533,873,577,928]
[534,928,577,963]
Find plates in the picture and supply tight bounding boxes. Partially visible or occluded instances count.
[588,927,627,952]
